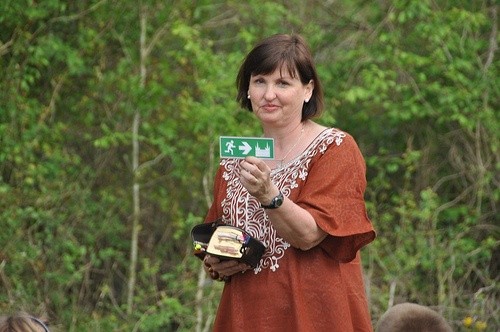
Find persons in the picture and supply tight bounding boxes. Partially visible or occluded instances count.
[191,32,376,332]
[0,312,49,332]
[375,303,454,332]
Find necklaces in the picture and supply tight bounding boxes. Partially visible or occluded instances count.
[275,122,306,162]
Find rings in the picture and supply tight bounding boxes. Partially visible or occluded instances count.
[253,179,258,185]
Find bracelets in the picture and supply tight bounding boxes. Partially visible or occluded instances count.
[204,255,230,282]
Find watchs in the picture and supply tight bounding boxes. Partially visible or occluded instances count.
[262,191,284,210]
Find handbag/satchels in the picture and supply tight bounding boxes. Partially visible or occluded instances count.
[191,217,265,268]
